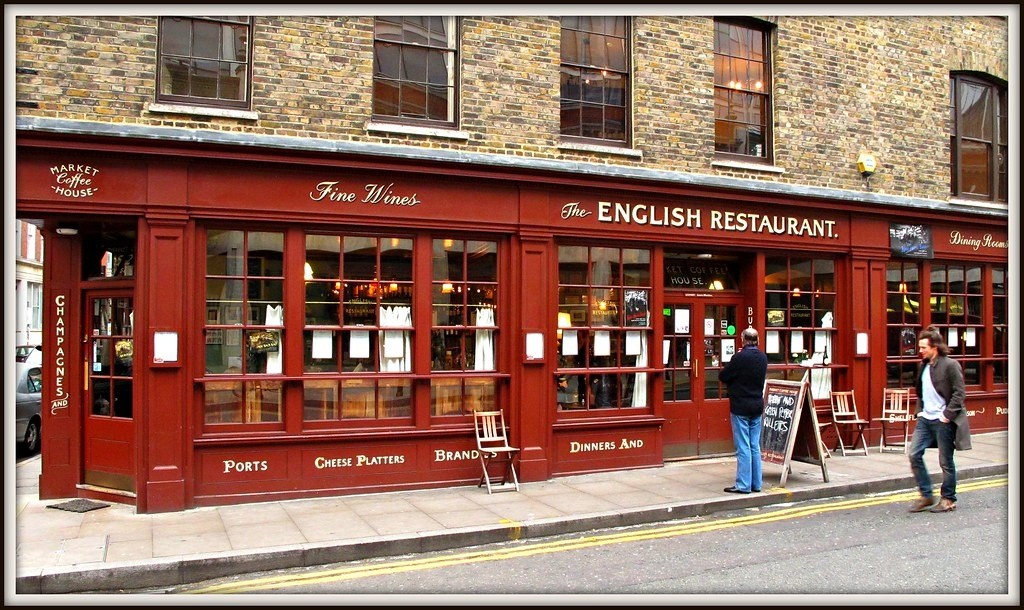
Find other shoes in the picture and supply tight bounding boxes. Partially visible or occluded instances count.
[909,496,940,512]
[723,486,750,494]
[929,497,956,513]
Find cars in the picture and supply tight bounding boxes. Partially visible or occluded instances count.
[16,345,35,363]
[16,362,42,457]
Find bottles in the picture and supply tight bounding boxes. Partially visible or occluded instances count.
[433,346,442,369]
[445,351,452,370]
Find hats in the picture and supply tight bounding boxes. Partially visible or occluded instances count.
[740,328,760,345]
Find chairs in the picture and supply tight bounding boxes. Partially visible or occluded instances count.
[819,387,911,457]
[473,408,522,495]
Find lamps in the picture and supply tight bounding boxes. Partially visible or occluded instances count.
[318,22,908,341]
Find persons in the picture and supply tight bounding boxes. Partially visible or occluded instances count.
[908,325,972,513]
[719,328,767,494]
